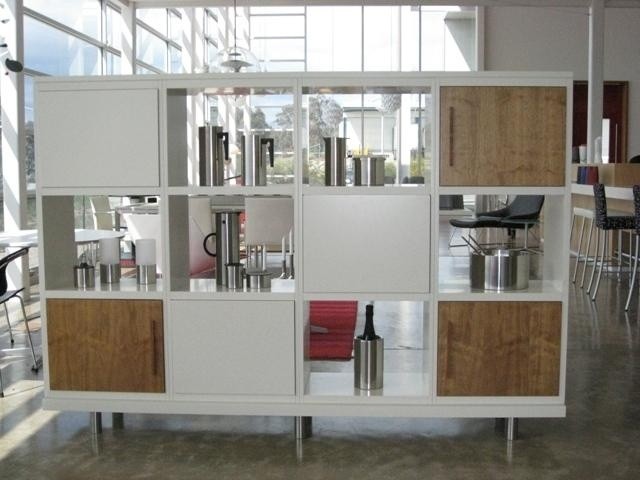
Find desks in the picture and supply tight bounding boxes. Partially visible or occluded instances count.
[2,227,124,257]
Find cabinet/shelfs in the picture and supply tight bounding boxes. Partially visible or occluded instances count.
[29,69,571,420]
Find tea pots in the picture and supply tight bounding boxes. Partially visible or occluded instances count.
[198,126,228,186]
[204,212,240,286]
[241,135,274,187]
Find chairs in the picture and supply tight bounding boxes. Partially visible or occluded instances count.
[591,182,635,302]
[572,204,632,285]
[1,247,41,398]
[448,192,546,251]
[624,185,640,311]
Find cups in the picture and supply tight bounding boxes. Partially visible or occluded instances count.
[578,146,587,164]
[226,263,242,289]
